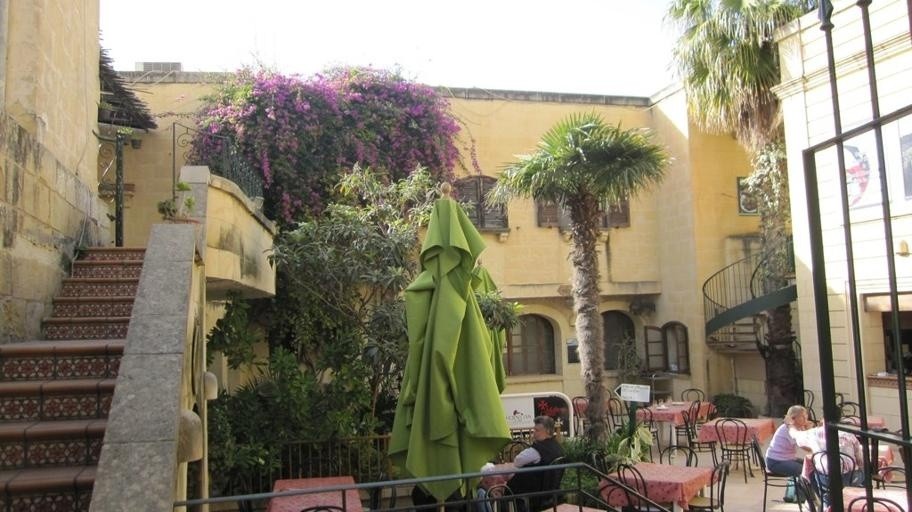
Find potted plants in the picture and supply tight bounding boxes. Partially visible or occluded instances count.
[96,100,112,121]
[157,181,200,242]
[130,131,142,149]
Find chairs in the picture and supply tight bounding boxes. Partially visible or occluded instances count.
[571,388,862,484]
[587,446,728,511]
[234,441,568,512]
[751,416,910,512]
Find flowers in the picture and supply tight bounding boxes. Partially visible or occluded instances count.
[117,127,132,135]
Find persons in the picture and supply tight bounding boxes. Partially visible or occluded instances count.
[500,415,567,512]
[765,405,822,511]
[783,402,866,509]
[411,474,493,512]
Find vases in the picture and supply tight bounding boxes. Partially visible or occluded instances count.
[121,134,130,145]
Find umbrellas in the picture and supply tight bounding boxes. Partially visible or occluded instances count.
[385,180,514,512]
